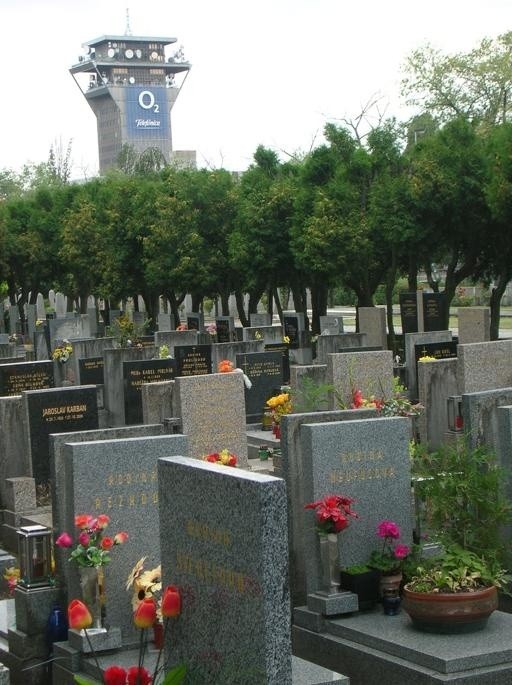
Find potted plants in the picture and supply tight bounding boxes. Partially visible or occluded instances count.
[403,562,508,635]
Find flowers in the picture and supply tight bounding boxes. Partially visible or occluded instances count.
[419,355,435,365]
[158,345,173,360]
[53,339,75,365]
[36,320,48,330]
[205,324,219,342]
[266,392,295,438]
[349,386,386,413]
[305,495,359,534]
[312,331,319,343]
[56,514,129,567]
[254,330,263,340]
[126,556,163,623]
[217,358,235,371]
[206,449,236,468]
[284,336,291,343]
[67,585,187,685]
[175,324,186,331]
[375,520,410,557]
[383,390,425,418]
[10,331,35,353]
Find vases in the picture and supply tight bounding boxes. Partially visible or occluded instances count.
[78,565,107,635]
[152,622,164,650]
[380,572,402,599]
[316,533,351,598]
[361,575,381,602]
